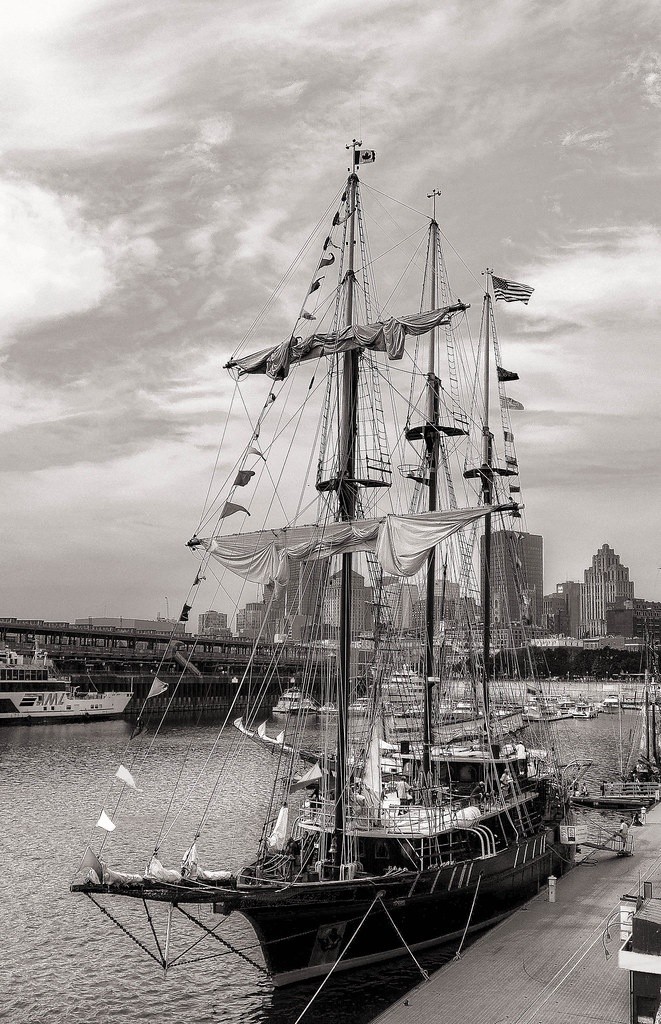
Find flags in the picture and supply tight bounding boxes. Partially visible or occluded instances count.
[491,274,535,305]
[497,365,534,625]
[355,150,375,164]
[80,191,352,885]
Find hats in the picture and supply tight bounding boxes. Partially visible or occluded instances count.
[518,741,523,744]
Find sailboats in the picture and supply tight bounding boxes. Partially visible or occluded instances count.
[64,128,585,1004]
[614,619,661,783]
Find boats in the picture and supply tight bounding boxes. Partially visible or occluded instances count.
[269,664,661,724]
[0,639,135,721]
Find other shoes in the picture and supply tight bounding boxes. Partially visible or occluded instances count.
[602,843,604,846]
[620,850,623,851]
[398,812,402,815]
[404,810,407,814]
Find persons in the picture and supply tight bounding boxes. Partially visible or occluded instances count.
[471,781,485,795]
[500,769,511,805]
[514,741,526,775]
[580,783,587,796]
[396,776,411,815]
[614,819,628,851]
